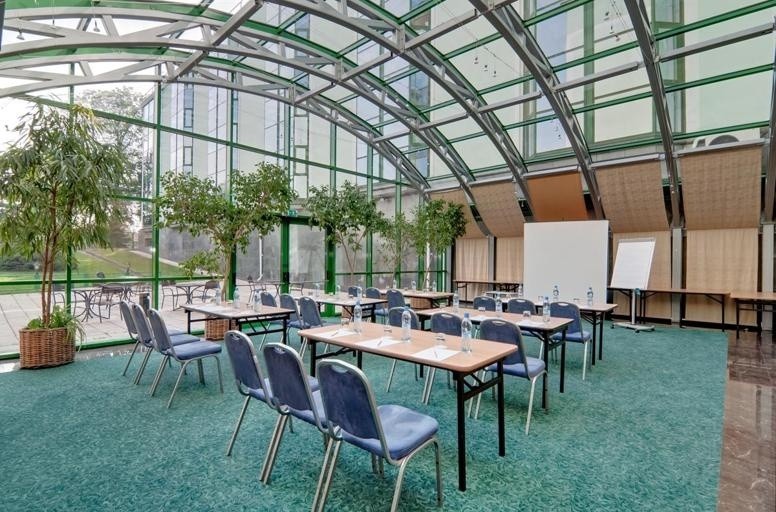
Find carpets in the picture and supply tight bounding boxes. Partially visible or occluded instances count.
[0,318,729,512]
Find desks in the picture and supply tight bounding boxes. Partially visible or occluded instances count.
[452,279,520,306]
[502,298,618,365]
[180,301,295,345]
[301,321,518,492]
[607,285,776,344]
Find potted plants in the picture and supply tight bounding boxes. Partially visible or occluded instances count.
[301,179,468,318]
[0,91,131,370]
[152,160,299,340]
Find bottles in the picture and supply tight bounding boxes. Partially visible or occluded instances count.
[353,300,363,333]
[459,311,471,352]
[399,306,412,341]
[208,285,263,313]
[453,284,594,323]
[308,279,438,301]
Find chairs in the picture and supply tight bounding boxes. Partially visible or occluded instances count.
[225,330,441,512]
[53,275,306,323]
[258,287,591,435]
[120,300,224,409]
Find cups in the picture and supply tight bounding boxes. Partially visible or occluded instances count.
[383,324,393,337]
[440,302,447,313]
[340,317,349,330]
[434,332,446,347]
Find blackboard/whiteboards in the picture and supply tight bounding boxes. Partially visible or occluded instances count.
[610,238,656,290]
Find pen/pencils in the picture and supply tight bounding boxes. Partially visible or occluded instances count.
[433,351,437,358]
[377,340,382,346]
[331,332,338,336]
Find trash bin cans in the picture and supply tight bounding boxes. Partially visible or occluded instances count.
[139,292,150,317]
